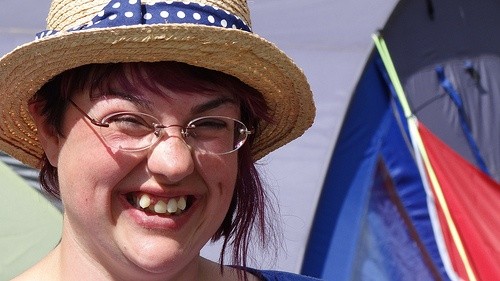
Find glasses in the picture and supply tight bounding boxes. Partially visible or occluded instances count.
[65,93,255,155]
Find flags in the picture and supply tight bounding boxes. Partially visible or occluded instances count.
[407,114,500,281]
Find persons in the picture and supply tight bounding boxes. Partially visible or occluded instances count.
[0,0,322,281]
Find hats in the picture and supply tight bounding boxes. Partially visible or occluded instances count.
[0,0,317,172]
